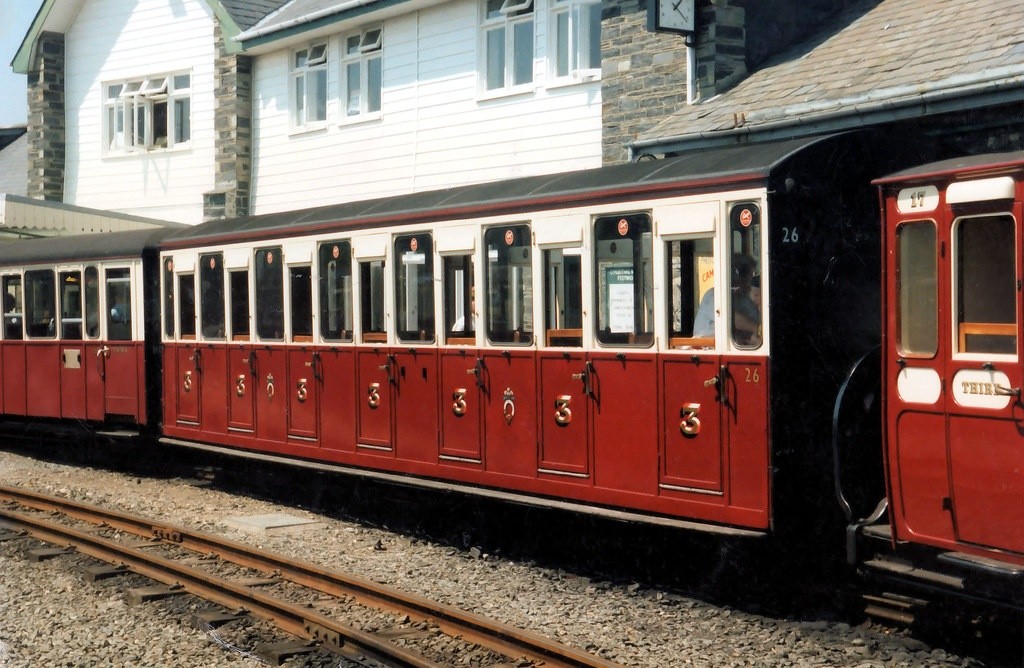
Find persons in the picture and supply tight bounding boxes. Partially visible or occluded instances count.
[452,287,475,331]
[693,253,763,344]
[79,285,130,337]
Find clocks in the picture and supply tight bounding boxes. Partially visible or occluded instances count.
[646,0,696,36]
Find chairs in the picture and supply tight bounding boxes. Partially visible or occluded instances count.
[182,328,715,349]
[959,322,1018,355]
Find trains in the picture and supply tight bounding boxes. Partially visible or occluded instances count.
[0,128,1024,663]
